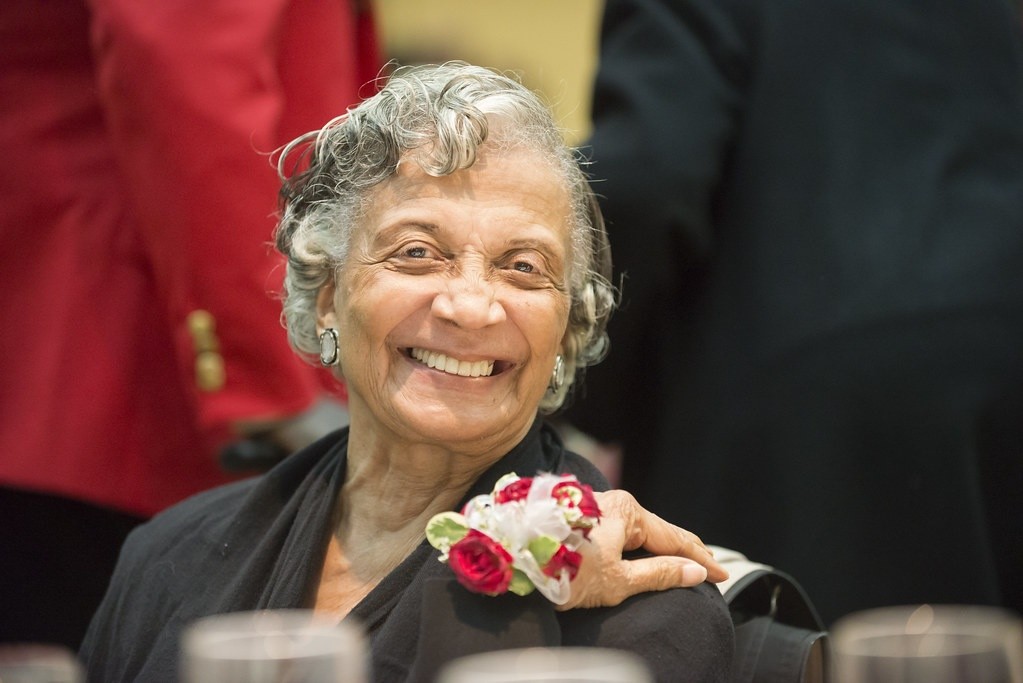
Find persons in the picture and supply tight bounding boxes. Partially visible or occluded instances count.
[77,59,738,683]
[0,0,390,659]
[565,0,1023,635]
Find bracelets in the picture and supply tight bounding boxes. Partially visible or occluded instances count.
[424,471,603,606]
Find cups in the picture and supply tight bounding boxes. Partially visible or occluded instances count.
[439,647,656,683]
[0,640,83,683]
[826,603,1023,683]
[178,609,369,683]
